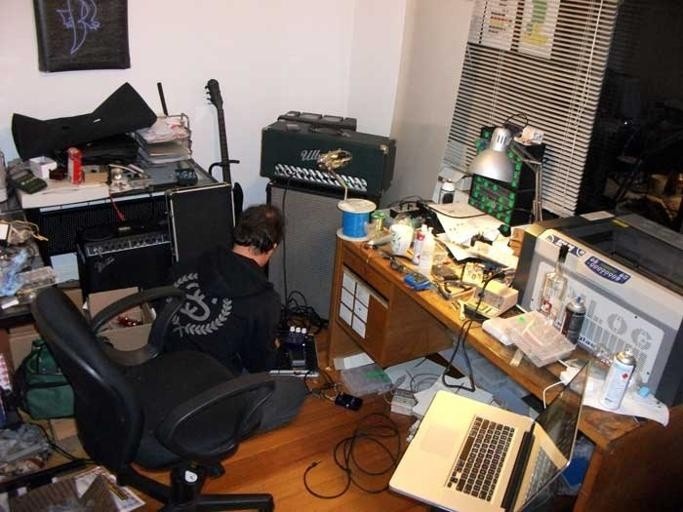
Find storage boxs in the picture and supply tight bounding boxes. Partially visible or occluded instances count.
[83,288,155,351]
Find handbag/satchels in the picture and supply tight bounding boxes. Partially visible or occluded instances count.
[12,339,75,421]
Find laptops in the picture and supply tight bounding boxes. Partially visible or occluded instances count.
[388,358,591,512]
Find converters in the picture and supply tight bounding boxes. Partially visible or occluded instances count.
[391,395,416,417]
[335,392,363,410]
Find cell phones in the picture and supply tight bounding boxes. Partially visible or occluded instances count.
[285,123,300,132]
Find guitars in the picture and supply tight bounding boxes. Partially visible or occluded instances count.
[205,78,244,228]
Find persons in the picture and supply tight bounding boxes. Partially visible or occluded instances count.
[146,204,307,435]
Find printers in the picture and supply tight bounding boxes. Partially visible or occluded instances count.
[512,210,683,407]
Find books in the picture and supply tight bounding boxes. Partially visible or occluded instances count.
[131,125,190,165]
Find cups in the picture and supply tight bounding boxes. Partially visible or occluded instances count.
[390,224,413,256]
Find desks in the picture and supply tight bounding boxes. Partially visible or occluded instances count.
[325,221,682,512]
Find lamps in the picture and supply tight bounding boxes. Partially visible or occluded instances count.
[466,126,545,224]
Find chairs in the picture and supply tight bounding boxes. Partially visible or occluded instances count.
[29,283,275,512]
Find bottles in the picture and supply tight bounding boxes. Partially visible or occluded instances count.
[559,296,585,346]
[598,349,636,410]
[539,244,570,322]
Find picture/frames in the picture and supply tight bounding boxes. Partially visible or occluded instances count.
[32,0,130,72]
